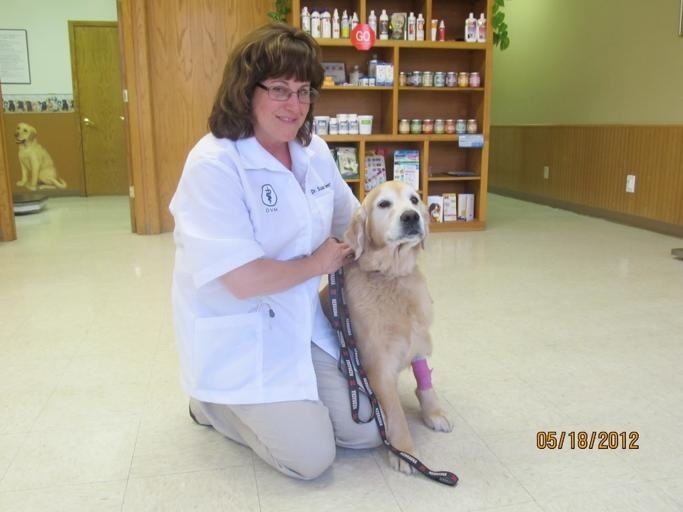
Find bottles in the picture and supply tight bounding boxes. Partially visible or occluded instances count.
[299,6,359,41]
[368,7,424,42]
[327,111,359,137]
[353,53,377,87]
[438,19,446,41]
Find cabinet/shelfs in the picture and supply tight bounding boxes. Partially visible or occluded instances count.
[290,0,493,231]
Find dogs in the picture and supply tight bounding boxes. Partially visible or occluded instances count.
[14,123,67,191]
[320,181,454,475]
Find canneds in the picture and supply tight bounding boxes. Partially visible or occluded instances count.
[398,117,478,135]
[400,70,482,88]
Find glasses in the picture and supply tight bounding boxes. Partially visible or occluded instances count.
[254,82,321,105]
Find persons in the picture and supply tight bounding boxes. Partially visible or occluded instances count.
[169,23,421,481]
